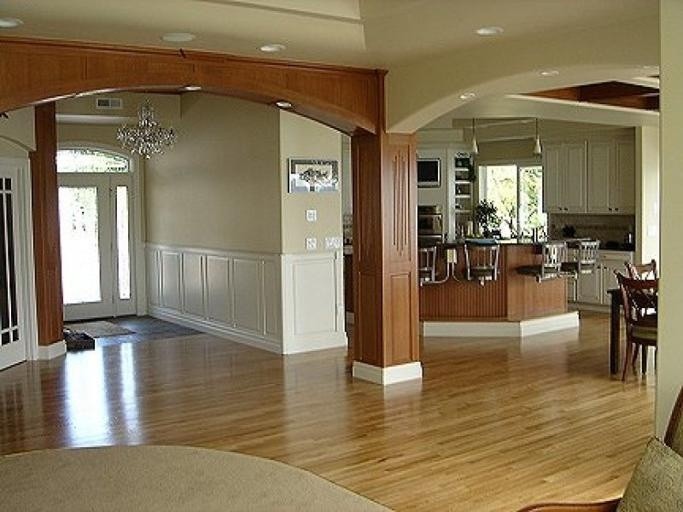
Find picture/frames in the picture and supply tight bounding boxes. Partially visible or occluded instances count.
[288,156,340,192]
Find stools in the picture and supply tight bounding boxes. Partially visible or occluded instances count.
[462,244,500,285]
[418,242,451,288]
[563,241,600,279]
[517,242,564,282]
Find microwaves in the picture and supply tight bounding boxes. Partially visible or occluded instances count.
[418,213,442,235]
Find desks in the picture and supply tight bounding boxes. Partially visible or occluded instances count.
[607,289,657,374]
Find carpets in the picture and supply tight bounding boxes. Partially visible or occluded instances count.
[0,445,397,512]
[63,321,134,337]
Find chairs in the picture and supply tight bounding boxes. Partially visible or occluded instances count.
[518,385,683,511]
[614,269,657,381]
[623,260,657,327]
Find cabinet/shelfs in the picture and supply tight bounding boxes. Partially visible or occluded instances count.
[543,142,586,213]
[452,154,475,238]
[568,249,633,312]
[587,141,636,216]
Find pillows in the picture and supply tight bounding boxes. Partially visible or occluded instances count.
[614,437,683,510]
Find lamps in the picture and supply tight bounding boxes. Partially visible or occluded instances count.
[116,91,178,162]
[470,118,479,155]
[532,118,542,155]
